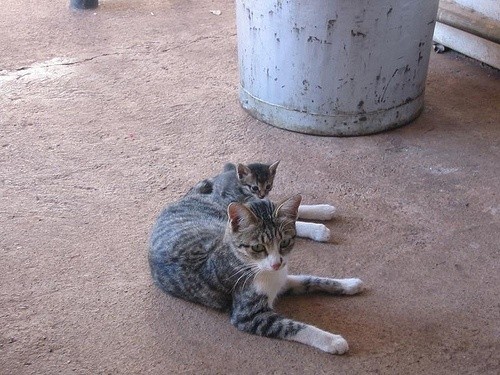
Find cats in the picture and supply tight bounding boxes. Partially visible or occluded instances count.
[146,160,366,354]
